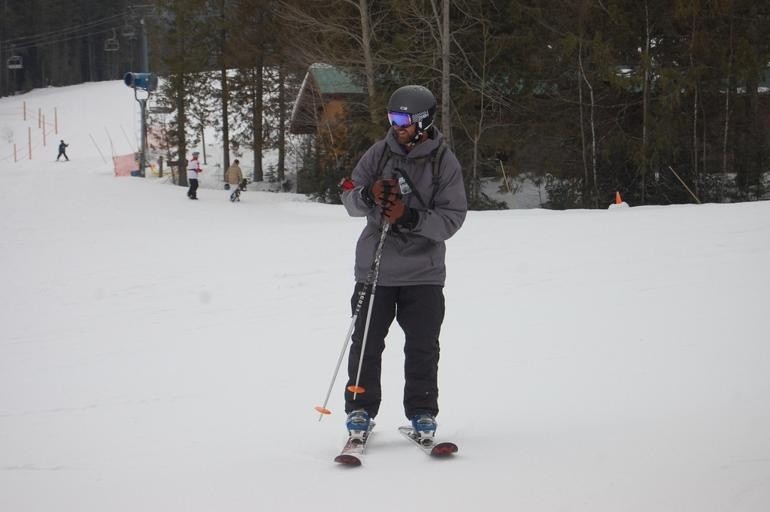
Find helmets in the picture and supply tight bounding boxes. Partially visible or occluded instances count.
[386,84,436,126]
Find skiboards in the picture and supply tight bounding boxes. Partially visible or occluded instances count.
[335,421,458,464]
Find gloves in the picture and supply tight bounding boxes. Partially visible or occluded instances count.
[375,197,408,222]
[363,176,400,209]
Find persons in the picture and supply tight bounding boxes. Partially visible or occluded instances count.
[329,82,469,450]
[224,159,244,202]
[185,152,203,200]
[56,140,71,161]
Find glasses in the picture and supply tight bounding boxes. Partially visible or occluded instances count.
[387,106,435,127]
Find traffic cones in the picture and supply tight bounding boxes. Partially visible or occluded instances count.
[616,190,623,205]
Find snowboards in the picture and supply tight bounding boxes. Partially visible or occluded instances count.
[230,179,247,201]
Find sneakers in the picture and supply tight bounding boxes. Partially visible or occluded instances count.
[346,409,371,430]
[410,412,438,430]
